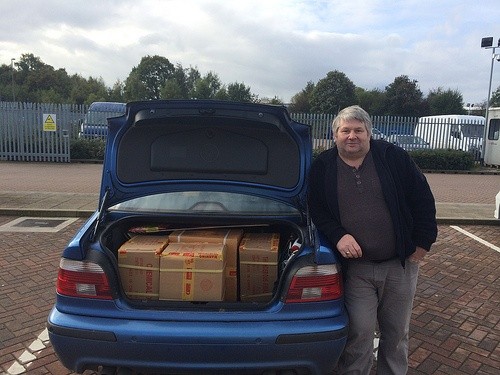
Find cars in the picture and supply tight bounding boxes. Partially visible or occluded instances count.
[370,128,387,141]
[45,99,350,375]
[387,134,429,154]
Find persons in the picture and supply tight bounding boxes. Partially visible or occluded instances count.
[306,106,437,375]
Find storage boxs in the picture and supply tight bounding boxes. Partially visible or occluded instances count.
[118,229,280,302]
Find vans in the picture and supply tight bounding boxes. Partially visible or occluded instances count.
[415,115,486,161]
[77,101,129,140]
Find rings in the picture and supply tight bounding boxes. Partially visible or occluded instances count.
[346,251,350,255]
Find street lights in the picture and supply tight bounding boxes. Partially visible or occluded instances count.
[480,37,500,164]
